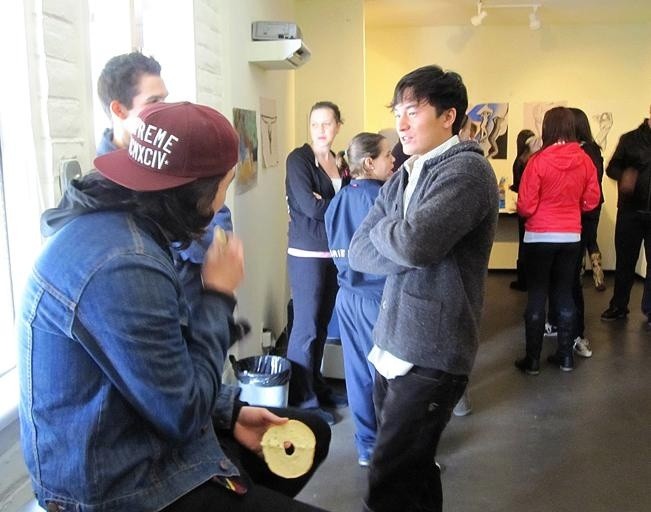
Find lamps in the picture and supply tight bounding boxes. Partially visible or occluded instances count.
[472,1,540,33]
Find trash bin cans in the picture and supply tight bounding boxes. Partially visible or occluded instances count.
[233,354,292,409]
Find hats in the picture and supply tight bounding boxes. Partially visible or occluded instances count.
[93,101,240,192]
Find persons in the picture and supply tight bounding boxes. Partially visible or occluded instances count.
[286,101,348,416]
[349,65,500,510]
[323,132,397,470]
[508,107,604,376]
[17,101,331,512]
[97,52,233,311]
[601,106,651,329]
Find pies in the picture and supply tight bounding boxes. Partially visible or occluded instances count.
[213,225,228,247]
[261,419,317,480]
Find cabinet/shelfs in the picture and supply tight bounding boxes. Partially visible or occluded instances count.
[488,208,518,273]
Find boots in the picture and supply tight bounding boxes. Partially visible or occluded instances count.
[510,260,527,291]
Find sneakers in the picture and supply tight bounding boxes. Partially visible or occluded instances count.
[600,308,627,321]
[302,395,349,426]
[516,322,593,375]
[453,385,472,417]
